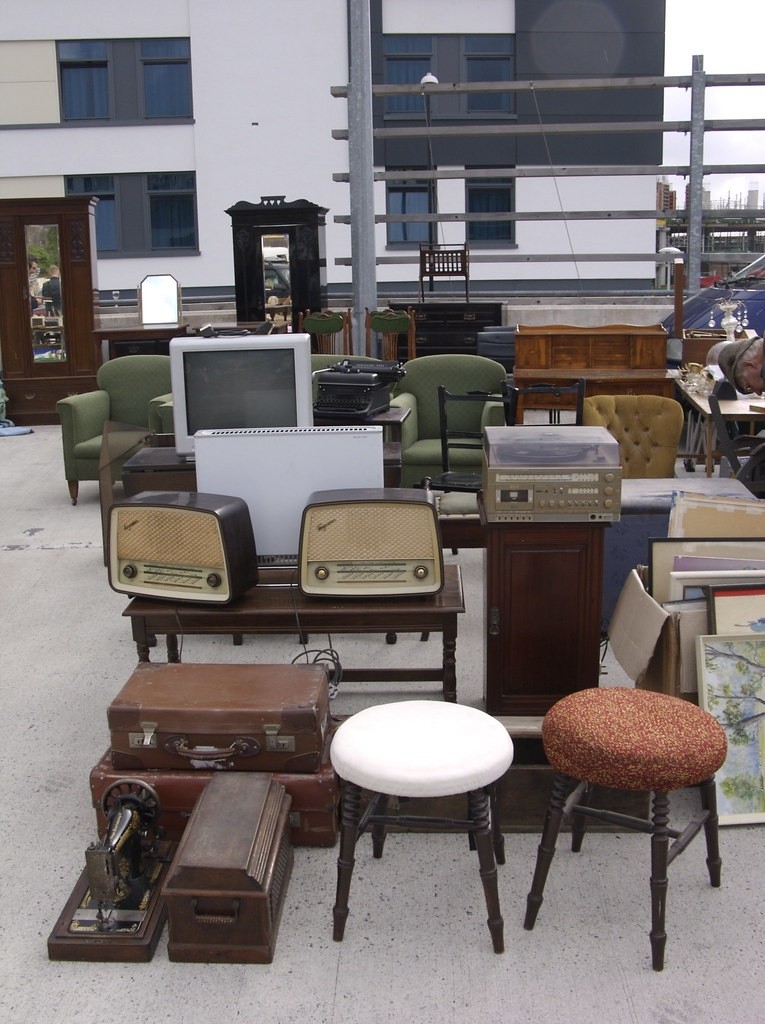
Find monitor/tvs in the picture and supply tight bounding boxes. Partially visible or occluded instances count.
[169,333,314,461]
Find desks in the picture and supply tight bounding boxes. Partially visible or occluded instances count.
[514,369,682,427]
[674,377,765,478]
[191,320,289,336]
[314,406,412,442]
[121,564,467,704]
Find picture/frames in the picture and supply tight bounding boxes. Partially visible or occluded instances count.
[704,583,765,635]
[693,633,765,827]
[668,570,765,602]
[647,539,765,677]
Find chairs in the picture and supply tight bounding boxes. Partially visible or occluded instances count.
[412,384,520,494]
[476,325,516,373]
[419,241,472,303]
[582,393,685,480]
[500,377,586,426]
[296,306,416,361]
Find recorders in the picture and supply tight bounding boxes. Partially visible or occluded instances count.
[103,490,259,605]
[298,488,445,597]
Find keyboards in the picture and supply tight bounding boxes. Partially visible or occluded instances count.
[313,398,369,412]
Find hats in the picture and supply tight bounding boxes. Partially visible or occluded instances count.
[718,337,761,396]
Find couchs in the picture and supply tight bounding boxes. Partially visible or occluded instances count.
[311,353,397,403]
[55,355,173,506]
[384,354,508,488]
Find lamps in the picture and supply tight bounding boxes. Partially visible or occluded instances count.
[708,287,749,341]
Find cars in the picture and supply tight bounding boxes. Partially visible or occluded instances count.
[662,253,765,344]
[264,256,290,305]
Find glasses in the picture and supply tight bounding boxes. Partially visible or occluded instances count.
[742,373,753,394]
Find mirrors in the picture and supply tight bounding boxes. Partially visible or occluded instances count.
[260,234,293,333]
[24,224,69,363]
[136,273,183,325]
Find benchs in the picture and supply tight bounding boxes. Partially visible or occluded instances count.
[599,477,758,636]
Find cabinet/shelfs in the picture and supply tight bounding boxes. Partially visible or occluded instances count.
[95,321,191,359]
[225,195,330,323]
[514,323,669,410]
[0,195,103,427]
[474,489,613,717]
[387,300,509,366]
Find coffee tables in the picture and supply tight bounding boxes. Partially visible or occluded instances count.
[121,441,403,497]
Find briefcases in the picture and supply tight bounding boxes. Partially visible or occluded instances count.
[89,737,341,850]
[106,658,332,774]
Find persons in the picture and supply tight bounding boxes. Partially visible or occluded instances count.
[718,336,765,396]
[29,257,61,316]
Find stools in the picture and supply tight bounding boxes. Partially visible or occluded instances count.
[523,686,727,973]
[330,700,506,956]
